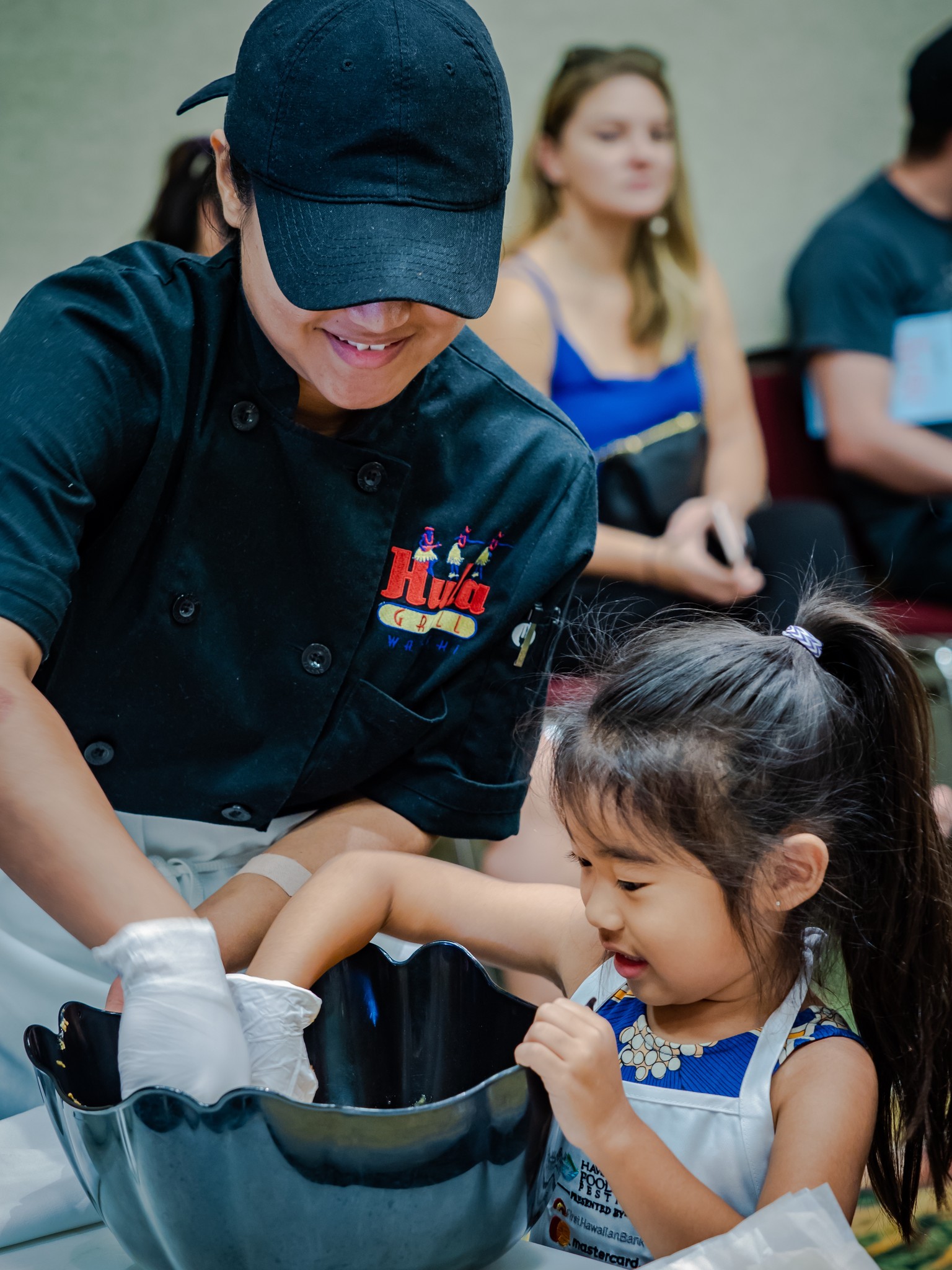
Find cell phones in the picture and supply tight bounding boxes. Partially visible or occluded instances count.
[706,508,744,569]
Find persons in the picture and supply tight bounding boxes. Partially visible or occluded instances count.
[792,19,951,596]
[225,598,951,1269]
[1,1,597,1118]
[465,40,865,677]
[141,136,242,258]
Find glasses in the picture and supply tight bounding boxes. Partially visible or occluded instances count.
[546,44,674,114]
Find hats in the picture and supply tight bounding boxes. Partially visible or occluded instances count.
[175,1,513,322]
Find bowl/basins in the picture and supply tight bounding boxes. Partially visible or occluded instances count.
[24,940,564,1270]
